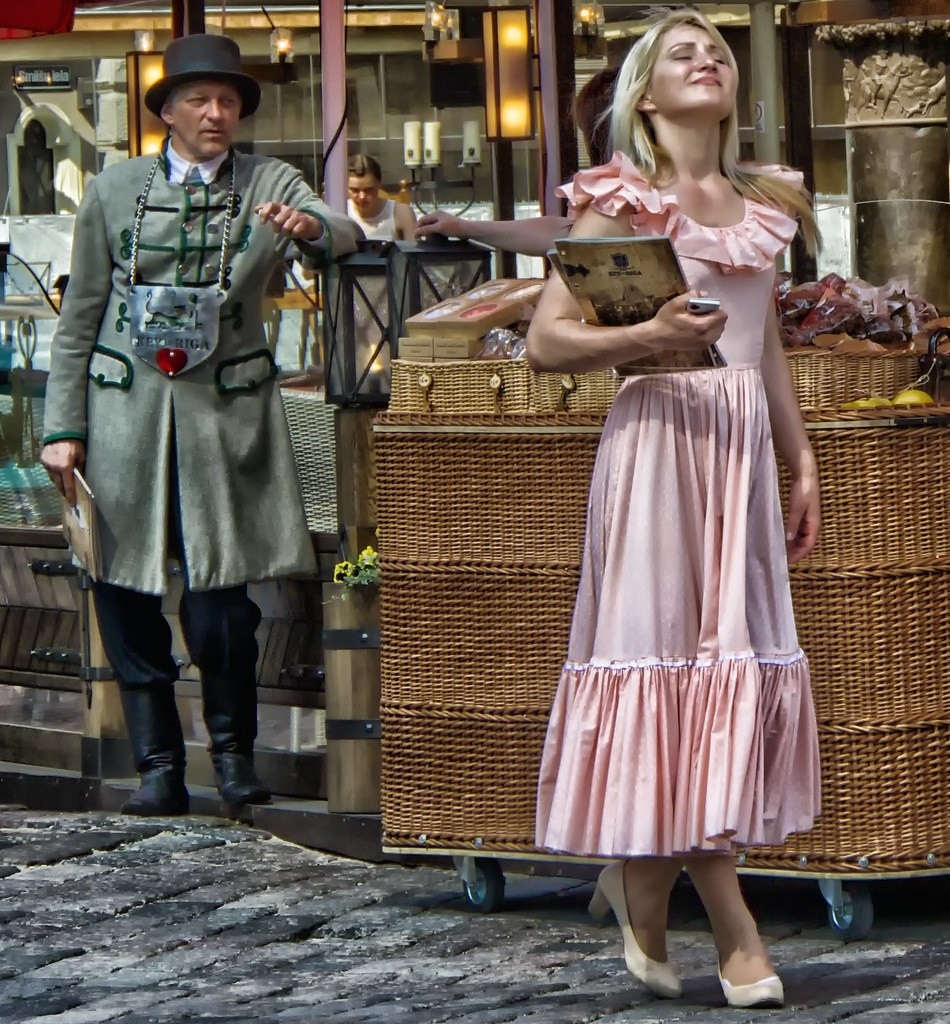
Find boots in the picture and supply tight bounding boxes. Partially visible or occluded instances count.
[119,684,191,818]
[200,666,258,806]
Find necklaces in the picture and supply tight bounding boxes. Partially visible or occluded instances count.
[124,148,236,375]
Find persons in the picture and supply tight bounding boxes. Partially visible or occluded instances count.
[41,32,365,814]
[415,68,622,257]
[302,154,422,280]
[525,9,820,1009]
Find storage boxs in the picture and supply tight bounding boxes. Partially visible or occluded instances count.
[397,277,549,364]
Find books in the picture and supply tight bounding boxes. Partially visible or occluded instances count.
[547,235,728,376]
[63,466,104,582]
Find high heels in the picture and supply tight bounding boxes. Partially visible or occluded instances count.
[587,860,682,999]
[717,953,786,1009]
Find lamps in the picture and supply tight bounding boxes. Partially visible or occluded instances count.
[321,236,495,402]
[260,27,298,85]
[126,50,164,161]
[421,1,452,42]
[134,29,153,52]
[572,1,606,60]
[482,5,540,142]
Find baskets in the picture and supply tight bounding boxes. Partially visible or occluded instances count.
[389,349,928,410]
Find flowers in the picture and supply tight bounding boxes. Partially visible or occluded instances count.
[320,547,381,605]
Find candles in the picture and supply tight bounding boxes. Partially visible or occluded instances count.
[403,121,423,164]
[422,122,440,164]
[462,121,480,163]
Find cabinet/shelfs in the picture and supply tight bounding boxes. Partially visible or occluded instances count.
[0,285,399,865]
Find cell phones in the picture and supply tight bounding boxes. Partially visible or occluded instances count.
[686,297,721,315]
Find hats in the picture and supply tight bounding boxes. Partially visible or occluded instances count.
[144,34,261,119]
